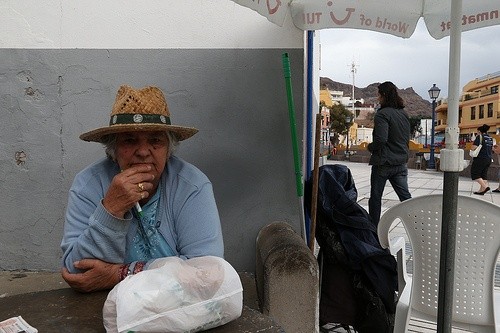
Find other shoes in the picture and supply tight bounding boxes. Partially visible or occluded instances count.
[474,192,484,195]
[492,189,500,193]
[479,187,490,194]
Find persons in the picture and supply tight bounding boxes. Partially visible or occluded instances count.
[492,184,500,193]
[60,89,224,292]
[471,124,493,195]
[365,82,412,220]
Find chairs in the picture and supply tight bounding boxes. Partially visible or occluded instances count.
[376,193,500,333]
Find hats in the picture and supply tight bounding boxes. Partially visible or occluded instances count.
[80,85,199,143]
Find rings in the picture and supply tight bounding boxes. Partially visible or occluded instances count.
[138,183,144,191]
[141,193,144,199]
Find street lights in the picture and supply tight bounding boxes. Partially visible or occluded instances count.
[428,84,441,169]
[346,116,352,153]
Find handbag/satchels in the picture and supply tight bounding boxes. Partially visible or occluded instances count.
[102,256,242,333]
[469,135,483,157]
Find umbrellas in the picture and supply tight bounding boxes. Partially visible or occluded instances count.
[230,0,500,333]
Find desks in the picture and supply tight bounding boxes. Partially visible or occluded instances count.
[0,286,286,333]
